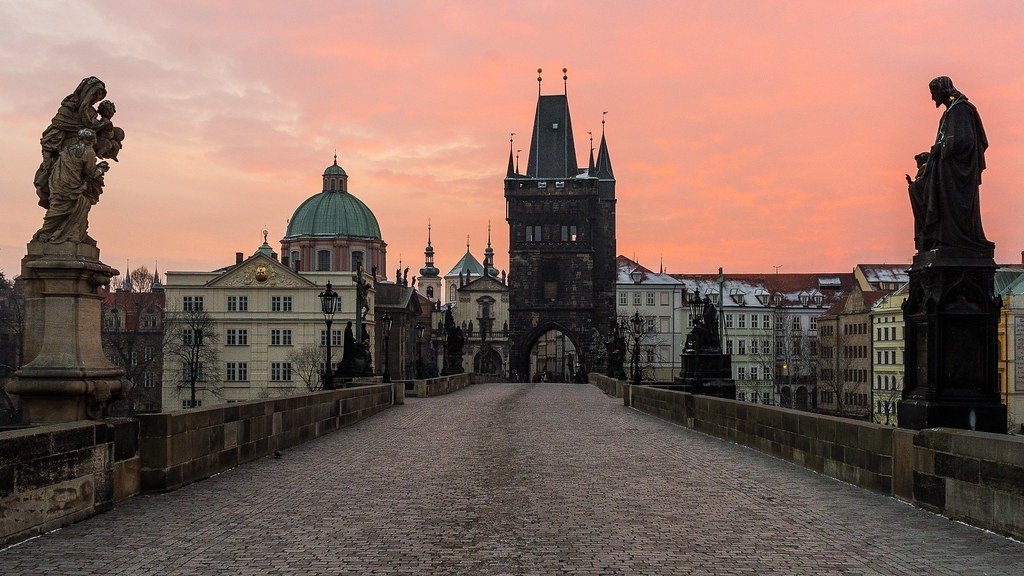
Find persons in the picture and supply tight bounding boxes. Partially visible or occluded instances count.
[30,75,125,245]
[905,74,997,253]
[360,283,371,321]
[462,320,467,336]
[502,320,509,336]
[360,323,370,343]
[396,266,416,287]
[685,297,719,349]
[437,319,443,335]
[340,321,356,360]
[468,320,474,336]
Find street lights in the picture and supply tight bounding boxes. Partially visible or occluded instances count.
[415,322,424,378]
[607,329,614,378]
[688,287,706,395]
[381,310,393,383]
[318,280,338,391]
[631,309,644,385]
[441,328,448,375]
